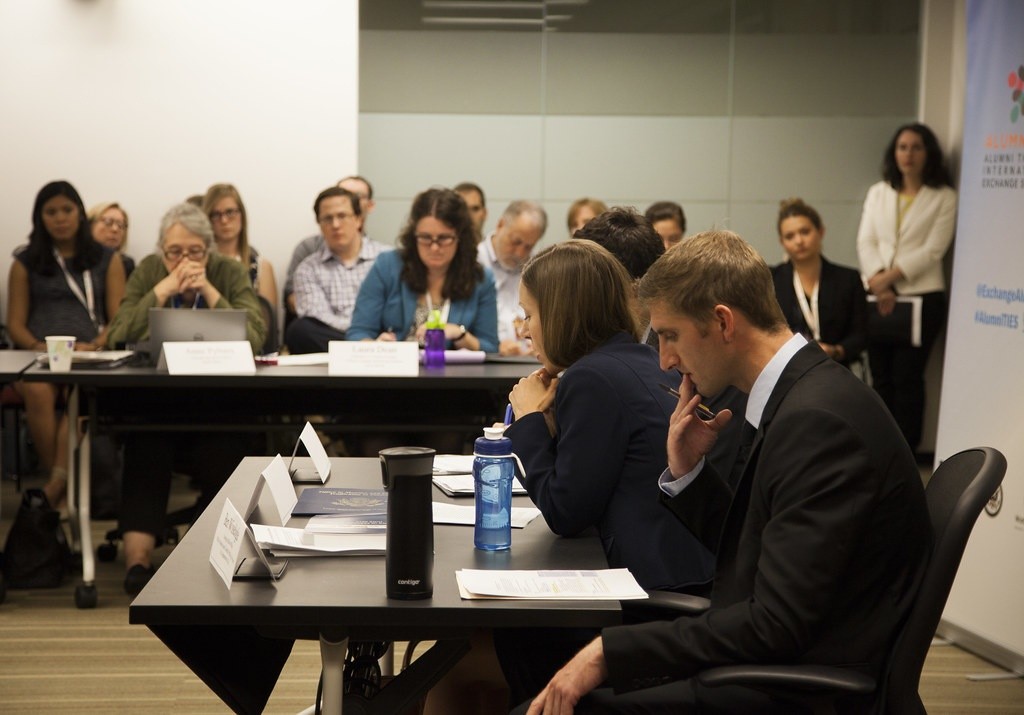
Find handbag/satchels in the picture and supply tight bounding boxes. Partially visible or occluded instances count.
[4,489,70,588]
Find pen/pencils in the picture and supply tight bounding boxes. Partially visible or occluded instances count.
[657,383,716,419]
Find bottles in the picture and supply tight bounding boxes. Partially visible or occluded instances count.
[379,446,435,600]
[422,309,446,371]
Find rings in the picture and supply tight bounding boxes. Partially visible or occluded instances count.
[192,275,197,281]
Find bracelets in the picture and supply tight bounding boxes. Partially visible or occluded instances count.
[30,340,40,350]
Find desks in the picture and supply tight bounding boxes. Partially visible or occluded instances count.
[0,344,624,715]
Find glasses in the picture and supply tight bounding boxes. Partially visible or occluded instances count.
[95,217,126,229]
[413,234,460,247]
[160,245,208,261]
[209,207,240,222]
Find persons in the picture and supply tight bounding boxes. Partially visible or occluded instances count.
[856,124,957,463]
[764,199,871,364]
[491,227,932,715]
[6,176,746,608]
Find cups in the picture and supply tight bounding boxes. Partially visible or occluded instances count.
[45,335,76,373]
[471,427,515,551]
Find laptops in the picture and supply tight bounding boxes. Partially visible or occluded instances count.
[146,307,247,367]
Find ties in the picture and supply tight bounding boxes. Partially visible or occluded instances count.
[739,420,757,474]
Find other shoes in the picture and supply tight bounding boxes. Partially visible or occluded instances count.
[124,562,156,595]
[41,481,66,508]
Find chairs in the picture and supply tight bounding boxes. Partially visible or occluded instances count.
[603,445,1007,715]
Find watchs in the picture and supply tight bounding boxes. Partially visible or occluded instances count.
[449,325,467,339]
